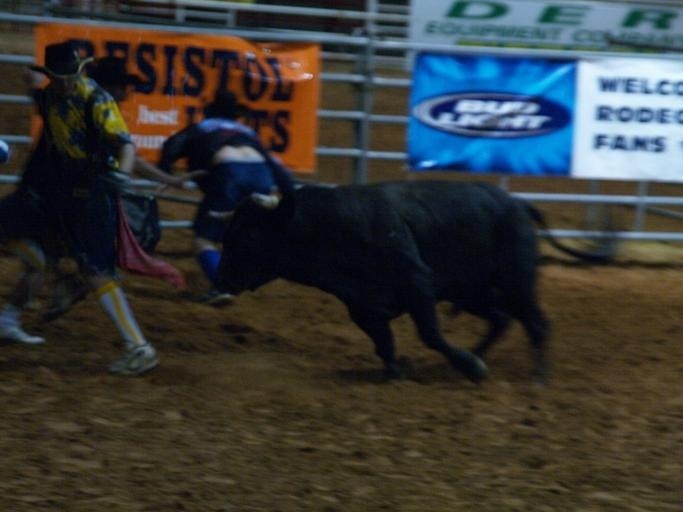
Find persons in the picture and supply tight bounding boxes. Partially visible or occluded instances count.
[10,43,205,376]
[158,88,296,307]
[1,180,90,342]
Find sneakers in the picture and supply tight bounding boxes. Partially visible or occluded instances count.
[106,338,161,377]
[0,325,46,346]
[199,286,232,306]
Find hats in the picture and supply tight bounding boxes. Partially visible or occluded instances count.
[85,54,145,86]
[28,42,95,81]
[203,90,249,117]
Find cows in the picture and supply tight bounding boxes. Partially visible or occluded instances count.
[207,177,613,387]
